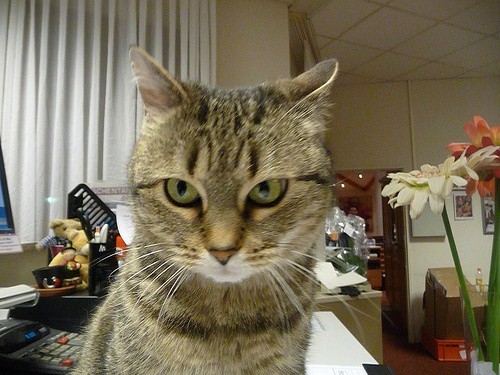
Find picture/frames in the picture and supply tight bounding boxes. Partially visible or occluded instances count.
[481,192,494,235]
[453,193,473,220]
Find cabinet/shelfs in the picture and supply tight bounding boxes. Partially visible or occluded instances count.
[369,246,382,289]
[315,290,383,365]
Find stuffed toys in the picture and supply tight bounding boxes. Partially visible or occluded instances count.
[34,219,90,291]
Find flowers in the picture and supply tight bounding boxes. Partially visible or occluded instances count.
[381,115,500,375]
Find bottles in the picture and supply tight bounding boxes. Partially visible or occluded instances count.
[476,268,483,294]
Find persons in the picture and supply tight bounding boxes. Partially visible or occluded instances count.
[349,205,370,232]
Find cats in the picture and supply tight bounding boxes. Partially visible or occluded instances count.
[68,45,391,375]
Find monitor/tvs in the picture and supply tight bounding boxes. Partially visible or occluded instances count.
[0,138,15,235]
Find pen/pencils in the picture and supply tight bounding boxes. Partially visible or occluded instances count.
[94,224,109,242]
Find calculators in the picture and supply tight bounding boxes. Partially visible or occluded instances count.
[0,320,86,372]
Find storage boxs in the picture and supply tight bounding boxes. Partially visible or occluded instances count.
[421,268,484,362]
